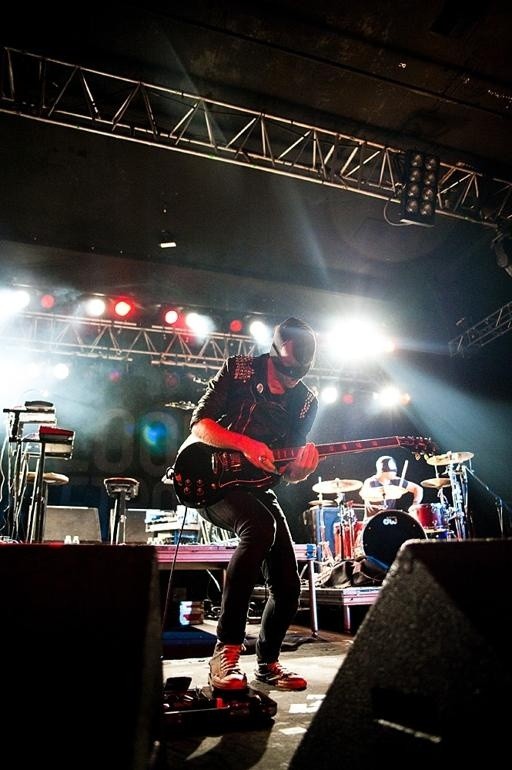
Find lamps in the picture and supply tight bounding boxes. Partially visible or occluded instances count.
[400,146,440,228]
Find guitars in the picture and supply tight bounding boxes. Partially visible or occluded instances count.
[172,435,434,507]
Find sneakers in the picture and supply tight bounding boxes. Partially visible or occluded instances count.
[254,664,306,691]
[209,642,247,689]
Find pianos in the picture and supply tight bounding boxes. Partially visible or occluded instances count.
[38,426,76,462]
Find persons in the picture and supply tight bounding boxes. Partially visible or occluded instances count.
[188,316,321,695]
[361,453,425,519]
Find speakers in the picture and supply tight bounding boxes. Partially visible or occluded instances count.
[287,538,512,770]
[0,545,164,770]
[42,506,103,542]
[110,509,151,544]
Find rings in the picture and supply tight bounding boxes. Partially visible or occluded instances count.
[258,455,270,464]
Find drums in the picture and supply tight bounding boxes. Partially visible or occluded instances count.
[355,510,428,555]
[332,522,363,562]
[410,504,442,529]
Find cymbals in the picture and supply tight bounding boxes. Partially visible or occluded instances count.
[366,485,402,502]
[425,451,473,466]
[307,498,335,505]
[419,478,450,490]
[311,480,362,493]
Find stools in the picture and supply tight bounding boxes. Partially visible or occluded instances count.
[101,477,139,545]
[22,471,70,543]
